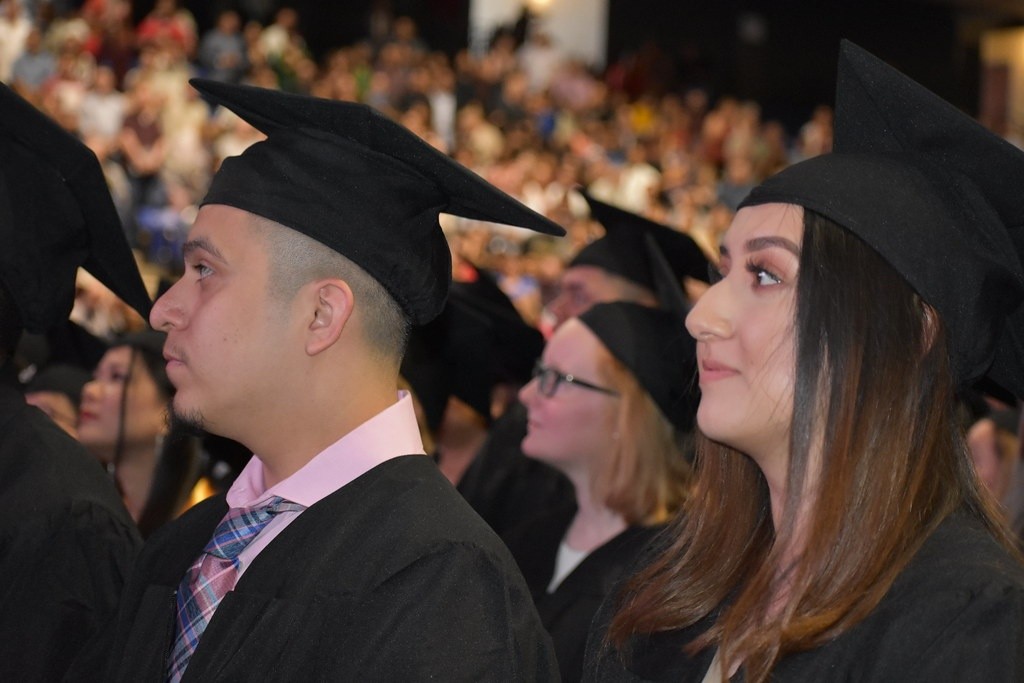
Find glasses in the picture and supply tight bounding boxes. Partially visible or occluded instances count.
[529,365,618,401]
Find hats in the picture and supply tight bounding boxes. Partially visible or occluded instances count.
[400,268,549,460]
[187,72,565,327]
[29,322,105,414]
[737,41,1024,402]
[113,280,168,359]
[576,301,699,434]
[562,184,719,304]
[0,86,154,320]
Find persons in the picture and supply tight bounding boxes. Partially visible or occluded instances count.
[2,87,154,683]
[77,327,228,593]
[582,38,1021,683]
[1,1,945,374]
[418,262,578,627]
[122,81,567,683]
[17,317,111,454]
[516,301,725,683]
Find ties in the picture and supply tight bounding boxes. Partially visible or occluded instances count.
[170,502,300,679]
